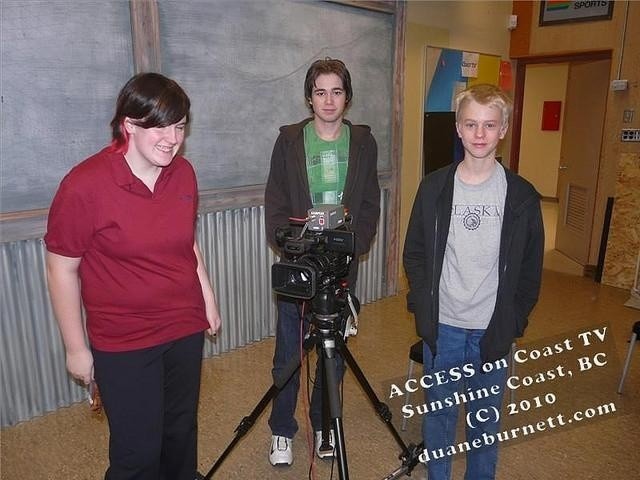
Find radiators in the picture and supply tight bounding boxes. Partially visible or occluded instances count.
[2,185,389,434]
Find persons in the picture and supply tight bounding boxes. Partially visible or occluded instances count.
[400,83,546,479]
[43,70,224,480]
[264,55,381,468]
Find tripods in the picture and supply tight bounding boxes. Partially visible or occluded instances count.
[204,313,428,480]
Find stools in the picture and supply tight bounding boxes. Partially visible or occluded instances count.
[615,320,639,395]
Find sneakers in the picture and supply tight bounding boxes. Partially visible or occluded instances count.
[314,429,337,460]
[268,435,294,467]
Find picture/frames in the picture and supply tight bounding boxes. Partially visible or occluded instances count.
[538,1,613,27]
